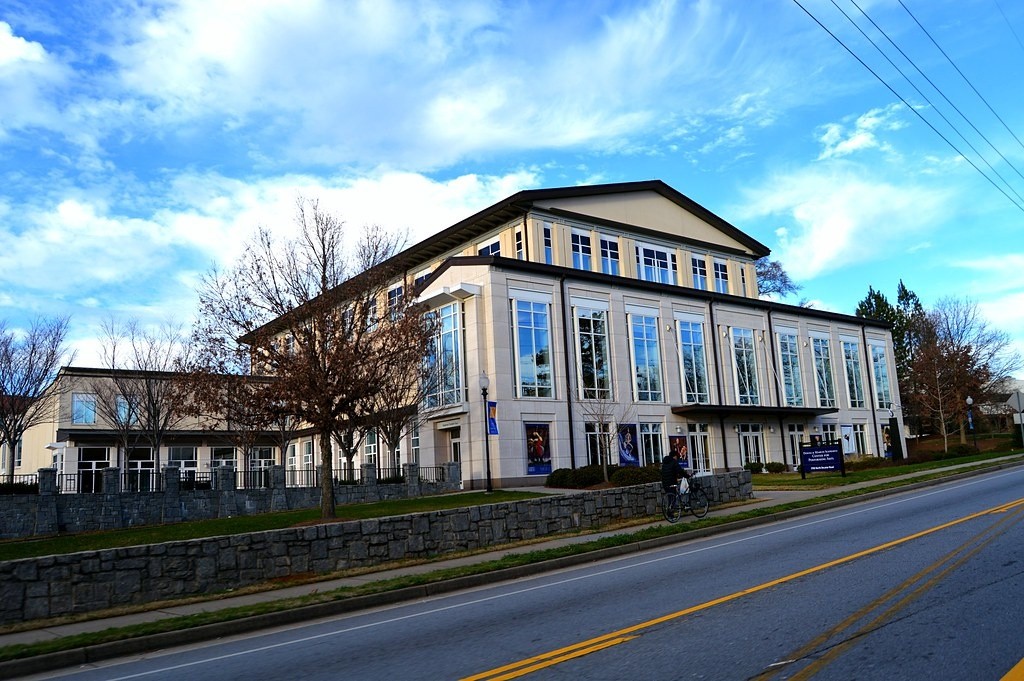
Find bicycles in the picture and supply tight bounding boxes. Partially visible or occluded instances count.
[661,470,710,523]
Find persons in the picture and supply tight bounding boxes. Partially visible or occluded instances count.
[674,438,682,453]
[680,446,687,461]
[661,450,692,521]
[530,432,544,463]
[624,426,633,455]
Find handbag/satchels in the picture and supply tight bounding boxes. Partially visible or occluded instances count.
[680,477,689,494]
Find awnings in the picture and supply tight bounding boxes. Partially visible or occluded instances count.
[671,404,840,415]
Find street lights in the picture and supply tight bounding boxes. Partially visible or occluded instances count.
[479,370,495,495]
[966,396,977,452]
